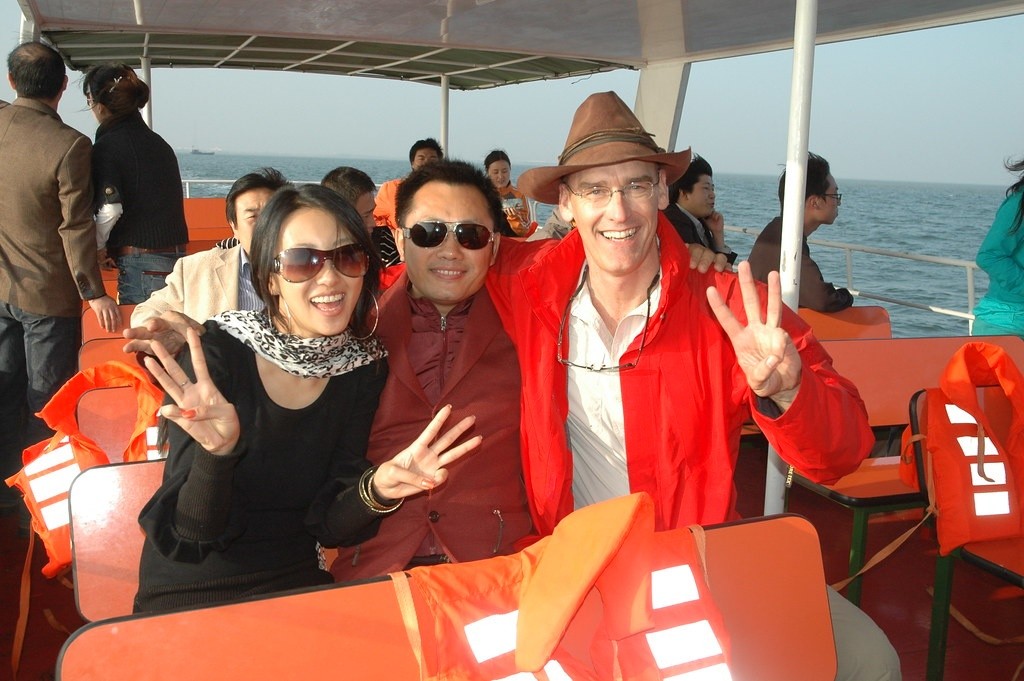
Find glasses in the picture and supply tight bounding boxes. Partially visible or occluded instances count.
[825,193,842,206]
[564,172,660,207]
[401,220,494,251]
[269,241,372,283]
[85,93,108,107]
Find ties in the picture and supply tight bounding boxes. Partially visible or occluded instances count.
[704,229,717,254]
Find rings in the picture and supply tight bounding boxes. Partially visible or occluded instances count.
[179,377,189,387]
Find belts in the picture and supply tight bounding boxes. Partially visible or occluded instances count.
[112,246,187,255]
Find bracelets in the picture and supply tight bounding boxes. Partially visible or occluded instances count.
[358,465,405,517]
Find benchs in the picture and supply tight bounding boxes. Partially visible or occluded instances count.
[57,199,1023,681]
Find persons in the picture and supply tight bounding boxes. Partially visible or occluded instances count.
[81,61,190,304]
[215,167,400,267]
[125,160,733,583]
[971,160,1024,338]
[381,90,876,552]
[523,206,577,241]
[130,172,295,330]
[0,42,123,539]
[663,155,738,264]
[132,184,483,614]
[747,152,854,313]
[374,138,443,230]
[484,150,531,235]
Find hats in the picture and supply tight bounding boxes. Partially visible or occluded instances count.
[517,91,692,206]
